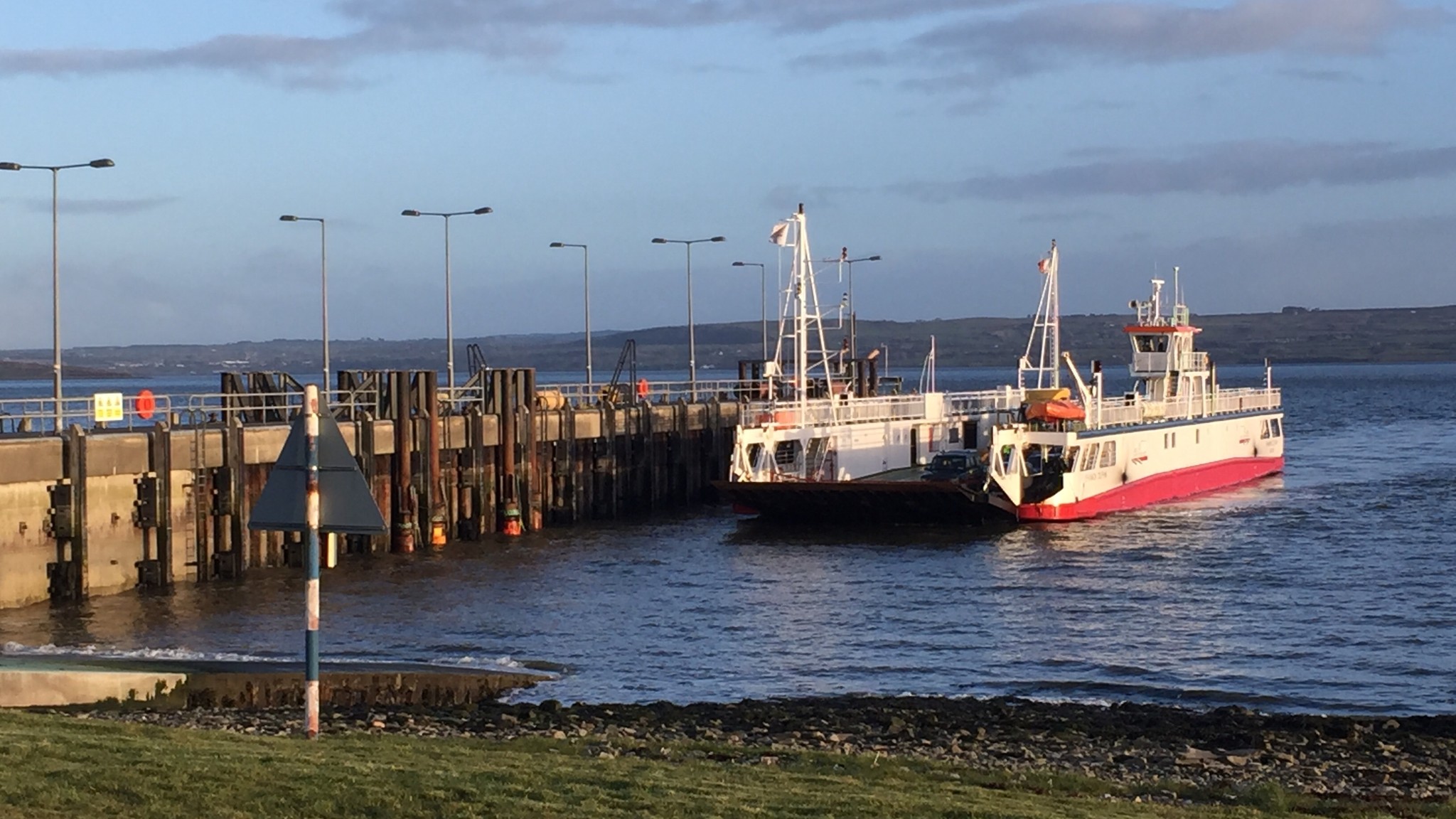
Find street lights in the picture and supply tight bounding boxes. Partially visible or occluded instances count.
[400,208,494,410]
[550,242,593,407]
[732,261,768,362]
[822,255,882,393]
[0,158,116,433]
[651,235,726,403]
[279,214,330,410]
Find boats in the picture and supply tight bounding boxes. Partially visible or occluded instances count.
[1024,397,1086,423]
[712,201,1287,523]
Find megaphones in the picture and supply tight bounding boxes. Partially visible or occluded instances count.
[1129,299,1138,309]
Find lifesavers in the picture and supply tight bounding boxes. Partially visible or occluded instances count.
[637,378,649,399]
[135,389,155,420]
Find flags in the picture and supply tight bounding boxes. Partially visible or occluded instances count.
[1037,259,1050,275]
[769,221,789,248]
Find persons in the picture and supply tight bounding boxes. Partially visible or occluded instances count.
[1142,340,1152,352]
[1157,337,1163,352]
[764,359,783,399]
